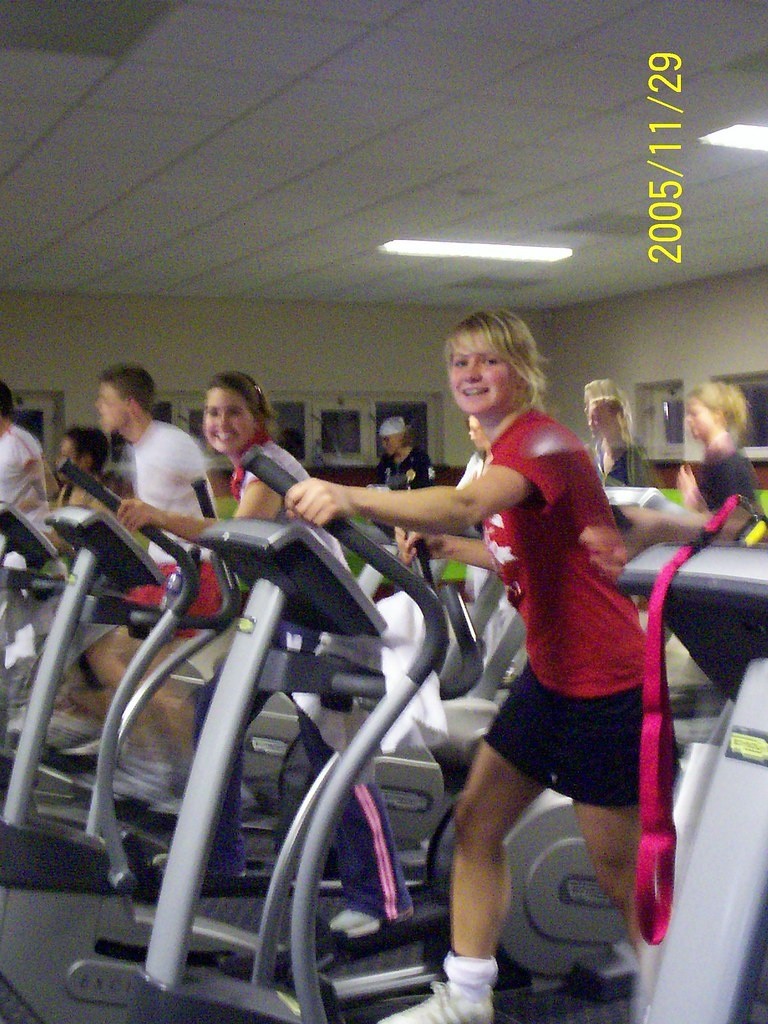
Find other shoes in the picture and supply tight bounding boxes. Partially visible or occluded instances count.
[8,703,260,815]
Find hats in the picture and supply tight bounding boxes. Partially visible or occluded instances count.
[378,417,405,437]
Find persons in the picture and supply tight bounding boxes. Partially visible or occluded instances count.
[119,371,417,942]
[363,414,442,548]
[61,361,233,764]
[19,423,154,700]
[584,378,663,489]
[1,385,69,676]
[673,380,765,543]
[449,415,522,682]
[283,306,677,1024]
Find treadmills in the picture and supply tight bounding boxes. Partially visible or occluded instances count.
[1,488,767,1024]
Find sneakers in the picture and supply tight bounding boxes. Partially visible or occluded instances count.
[151,854,246,877]
[377,982,494,1024]
[328,906,413,939]
